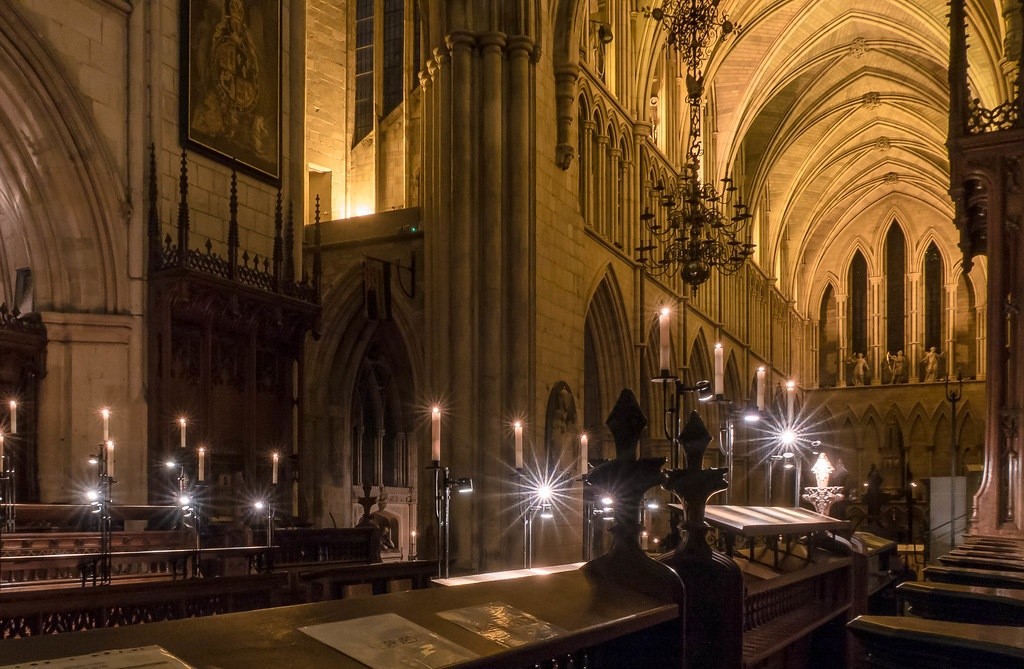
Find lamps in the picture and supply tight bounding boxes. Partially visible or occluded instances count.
[449,477,473,492]
[637,0,756,293]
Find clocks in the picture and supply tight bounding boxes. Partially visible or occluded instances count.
[199,447,204,481]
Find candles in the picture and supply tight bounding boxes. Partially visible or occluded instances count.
[108,440,114,476]
[659,308,670,370]
[715,343,724,395]
[0,432,3,477]
[104,409,109,441]
[515,421,524,469]
[432,407,442,463]
[273,453,278,484]
[756,367,766,411]
[787,381,795,424]
[11,401,17,433]
[181,418,186,447]
[581,433,589,475]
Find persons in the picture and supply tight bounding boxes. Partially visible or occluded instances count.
[848,346,945,385]
[380,525,394,551]
